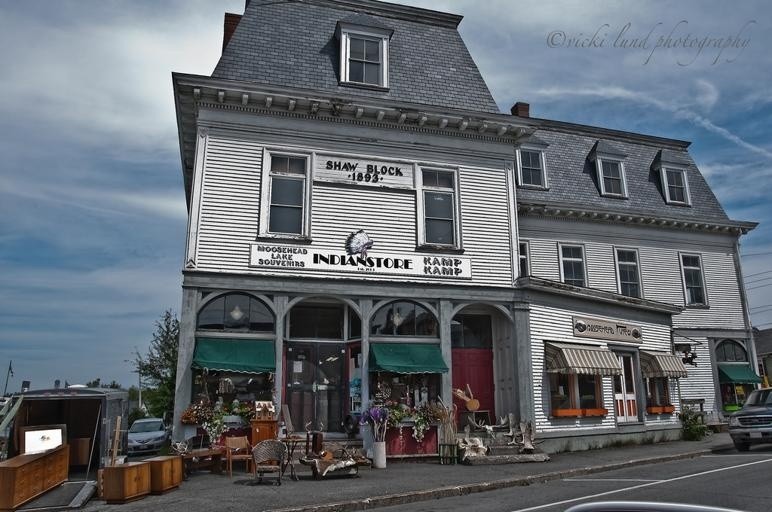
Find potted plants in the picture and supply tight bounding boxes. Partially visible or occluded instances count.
[676,406,712,441]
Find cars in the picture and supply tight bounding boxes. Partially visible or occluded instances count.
[128,418,168,457]
[728,387,772,451]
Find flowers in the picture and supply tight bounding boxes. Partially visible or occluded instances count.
[360,407,389,442]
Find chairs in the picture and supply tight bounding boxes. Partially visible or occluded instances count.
[225,435,286,486]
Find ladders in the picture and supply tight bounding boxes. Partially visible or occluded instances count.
[0,394,24,432]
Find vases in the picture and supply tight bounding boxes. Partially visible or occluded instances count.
[372,441,386,468]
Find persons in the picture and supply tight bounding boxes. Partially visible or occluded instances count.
[724,385,733,405]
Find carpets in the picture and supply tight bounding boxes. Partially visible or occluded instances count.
[19,483,86,509]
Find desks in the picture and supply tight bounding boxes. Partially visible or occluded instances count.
[281,437,371,480]
[461,409,491,431]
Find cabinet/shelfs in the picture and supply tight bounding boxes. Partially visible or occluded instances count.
[0,444,70,512]
[105,455,183,504]
[249,418,279,464]
